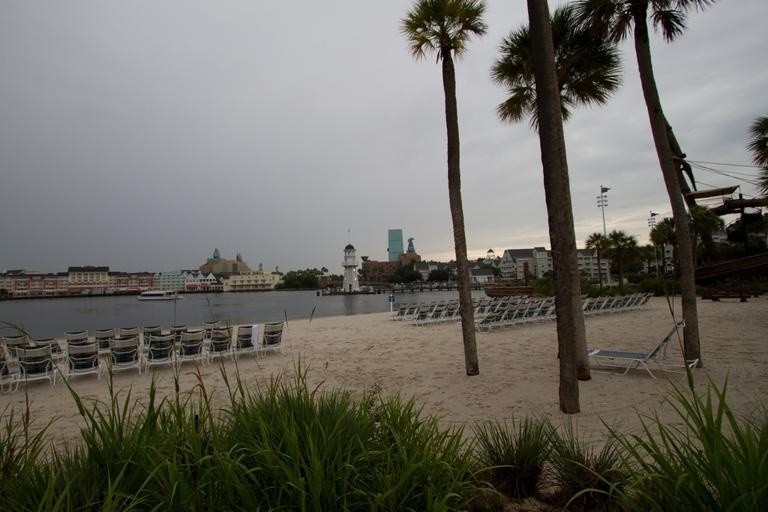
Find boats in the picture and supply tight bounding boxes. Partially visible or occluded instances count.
[136,289,186,302]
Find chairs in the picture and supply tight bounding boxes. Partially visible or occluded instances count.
[587,319,686,380]
[0,318,284,395]
[390,291,659,335]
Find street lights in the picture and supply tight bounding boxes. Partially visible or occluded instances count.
[647,217,663,278]
[596,191,611,285]
